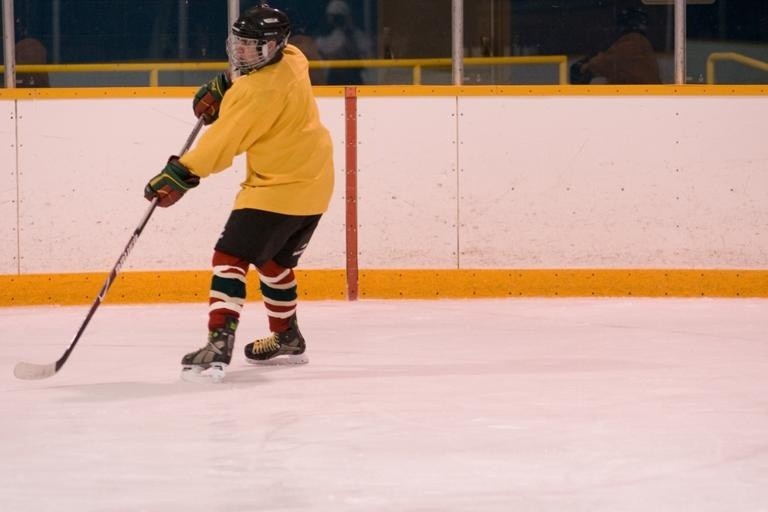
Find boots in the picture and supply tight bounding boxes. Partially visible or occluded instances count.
[245,316,306,360]
[182,318,238,370]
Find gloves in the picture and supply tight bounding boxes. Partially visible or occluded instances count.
[192,71,232,125]
[144,155,200,207]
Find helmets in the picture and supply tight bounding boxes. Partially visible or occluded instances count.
[227,8,292,73]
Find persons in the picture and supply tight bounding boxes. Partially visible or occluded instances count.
[572,0,661,83]
[319,0,375,80]
[287,6,325,83]
[16,13,52,89]
[144,2,336,366]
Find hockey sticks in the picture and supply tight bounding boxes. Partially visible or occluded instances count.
[14,112,207,380]
